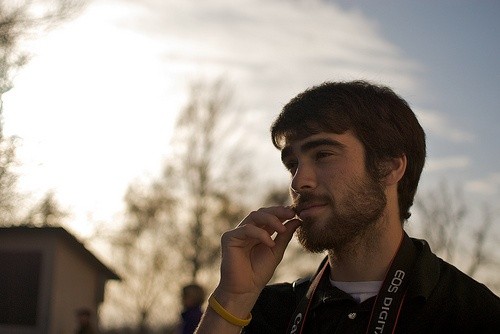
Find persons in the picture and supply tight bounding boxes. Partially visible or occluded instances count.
[182,284,206,334]
[195,80,500,334]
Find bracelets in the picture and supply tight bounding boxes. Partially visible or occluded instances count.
[208,295,252,326]
